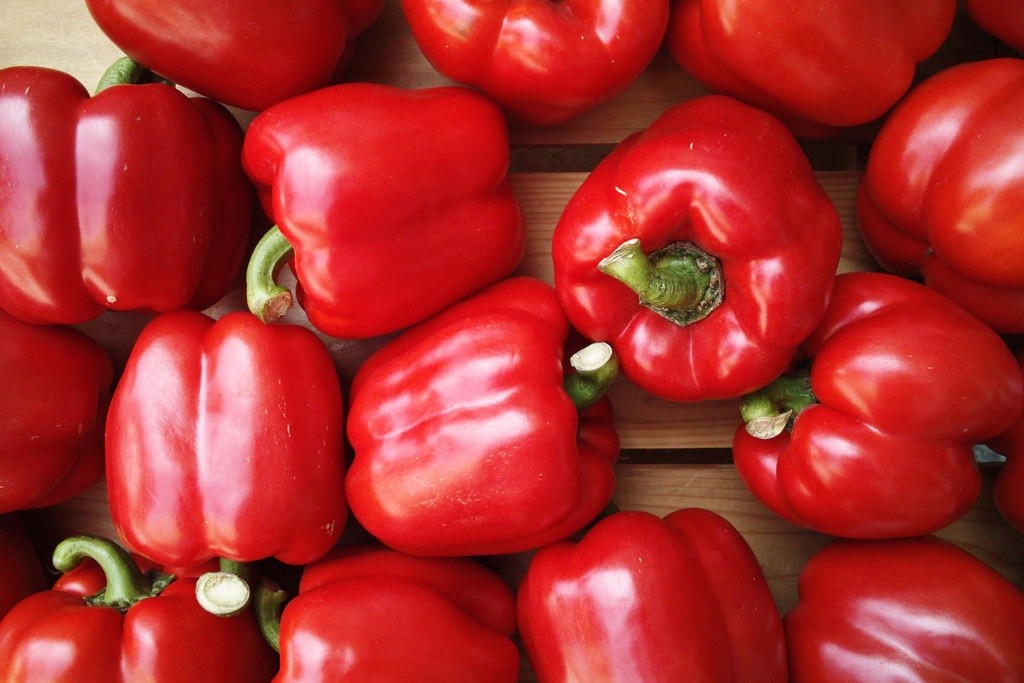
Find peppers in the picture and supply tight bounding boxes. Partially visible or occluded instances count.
[0,0,1024,683]
